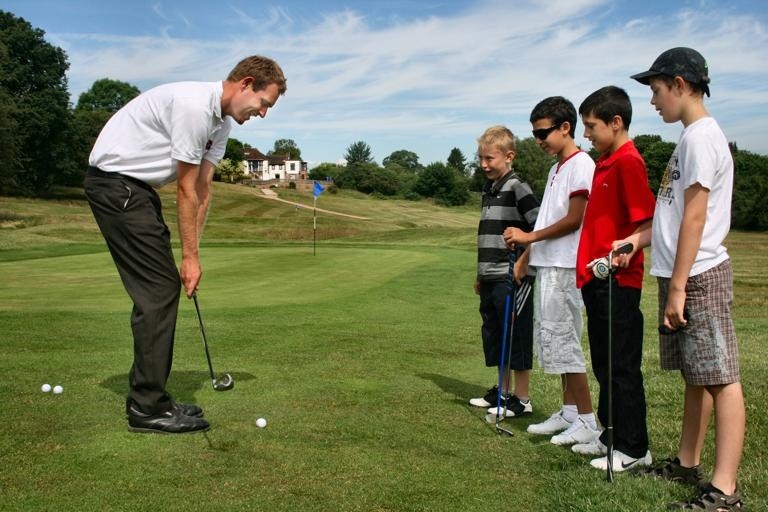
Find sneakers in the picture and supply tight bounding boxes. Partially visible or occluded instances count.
[470,384,511,407]
[550,415,600,445]
[642,457,703,485]
[486,395,532,417]
[527,410,573,435]
[667,483,743,512]
[590,449,652,472]
[572,437,607,455]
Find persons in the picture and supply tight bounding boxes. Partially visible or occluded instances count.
[468,125,537,420]
[501,97,596,448]
[609,47,748,512]
[571,83,654,472]
[84,55,288,436]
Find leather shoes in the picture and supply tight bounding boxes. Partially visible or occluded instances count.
[128,407,210,435]
[172,403,204,418]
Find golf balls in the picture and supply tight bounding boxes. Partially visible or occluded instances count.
[40,382,51,393]
[52,384,66,394]
[253,418,268,429]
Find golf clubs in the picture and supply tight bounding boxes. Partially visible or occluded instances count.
[486,286,518,425]
[495,241,516,438]
[604,242,637,483]
[189,293,234,389]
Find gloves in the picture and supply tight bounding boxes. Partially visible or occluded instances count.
[587,255,617,280]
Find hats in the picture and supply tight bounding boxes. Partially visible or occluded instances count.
[629,47,710,97]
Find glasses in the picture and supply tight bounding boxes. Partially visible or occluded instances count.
[532,125,560,140]
[658,312,690,335]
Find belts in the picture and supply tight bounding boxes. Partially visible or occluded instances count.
[87,166,125,179]
